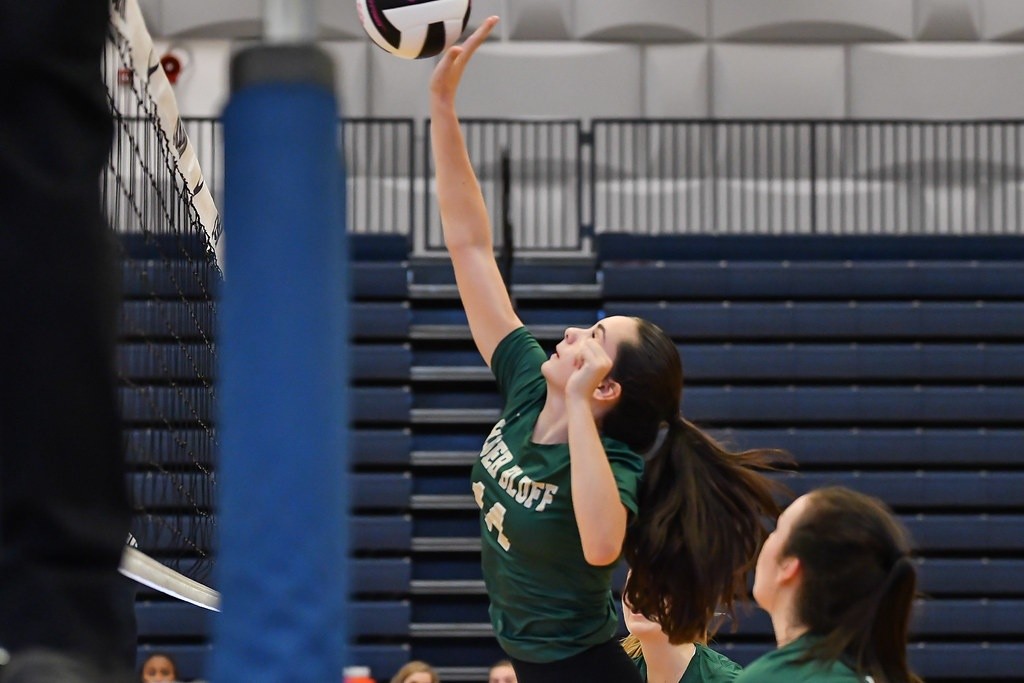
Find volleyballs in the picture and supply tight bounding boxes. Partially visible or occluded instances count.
[355,0,473,62]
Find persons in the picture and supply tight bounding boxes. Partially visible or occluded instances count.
[429,15,779,683]
[141,487,915,683]
